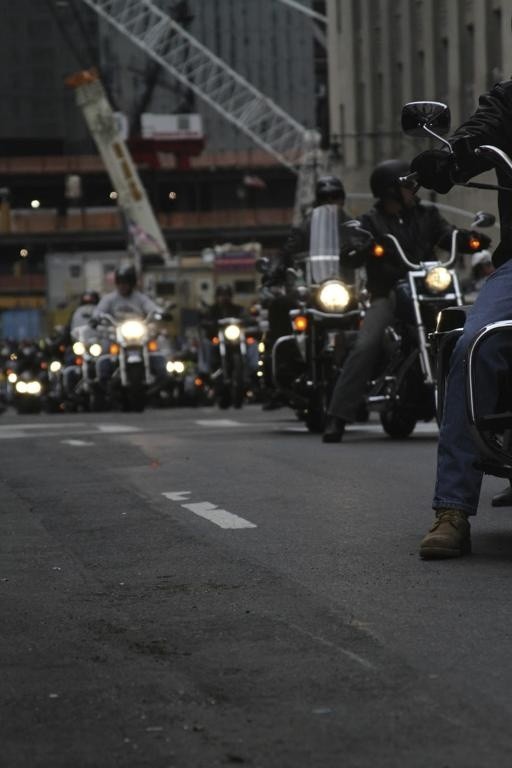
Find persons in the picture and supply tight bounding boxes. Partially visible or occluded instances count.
[1,263,286,415]
[259,173,362,416]
[320,159,493,442]
[462,247,494,303]
[408,73,512,559]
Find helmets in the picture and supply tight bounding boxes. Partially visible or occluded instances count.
[116,265,135,286]
[369,160,414,196]
[315,175,345,204]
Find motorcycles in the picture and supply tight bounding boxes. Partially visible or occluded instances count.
[1,280,213,415]
[254,204,377,433]
[195,282,259,411]
[343,207,496,447]
[395,100,512,486]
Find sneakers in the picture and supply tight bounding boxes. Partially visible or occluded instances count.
[323,416,345,441]
[420,509,472,558]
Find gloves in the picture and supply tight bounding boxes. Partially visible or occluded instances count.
[411,149,453,193]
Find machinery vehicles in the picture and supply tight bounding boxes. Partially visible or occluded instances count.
[31,0,264,341]
